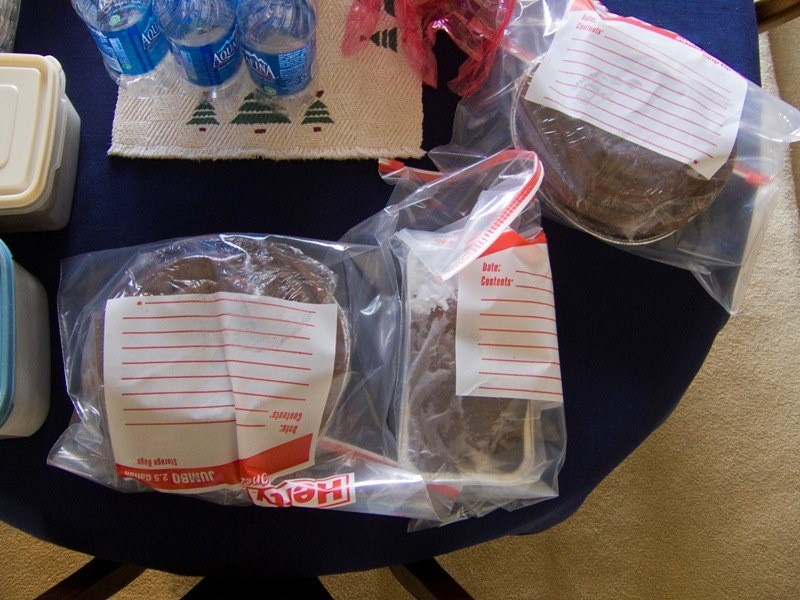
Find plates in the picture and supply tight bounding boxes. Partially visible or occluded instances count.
[511,54,695,245]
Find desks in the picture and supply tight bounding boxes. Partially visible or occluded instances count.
[0,0,762,581]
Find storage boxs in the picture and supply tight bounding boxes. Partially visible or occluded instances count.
[0,237,51,440]
[1,52,81,232]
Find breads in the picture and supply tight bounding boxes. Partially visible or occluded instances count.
[526,54,742,236]
[421,285,524,465]
[94,247,350,428]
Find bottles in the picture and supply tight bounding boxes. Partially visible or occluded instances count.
[235,0,320,108]
[153,0,243,100]
[71,0,176,96]
